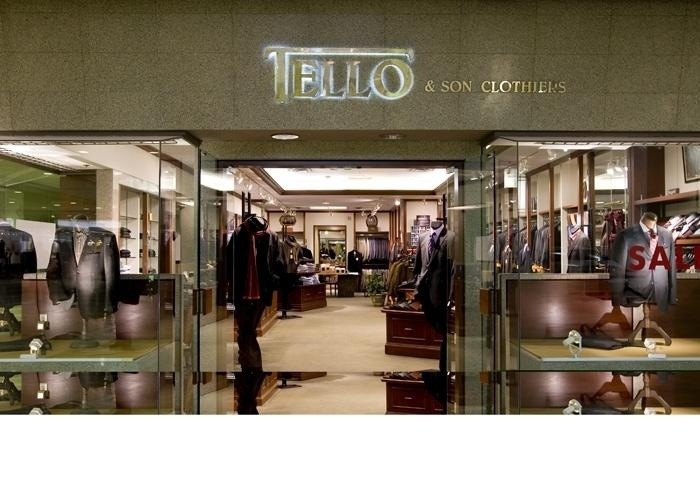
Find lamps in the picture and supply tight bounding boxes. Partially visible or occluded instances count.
[369,198,386,218]
[236,169,283,208]
[489,148,556,189]
[606,156,625,175]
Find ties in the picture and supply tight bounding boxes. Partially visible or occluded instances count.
[649,229,657,254]
[427,232,437,256]
[76,237,81,265]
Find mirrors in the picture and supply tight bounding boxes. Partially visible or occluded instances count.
[593,150,627,270]
[313,224,347,269]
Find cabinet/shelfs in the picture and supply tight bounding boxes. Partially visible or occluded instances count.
[277,283,326,311]
[140,219,159,259]
[636,190,700,246]
[382,378,445,415]
[118,214,138,260]
[379,308,442,360]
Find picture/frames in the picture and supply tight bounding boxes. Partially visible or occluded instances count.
[682,144,700,183]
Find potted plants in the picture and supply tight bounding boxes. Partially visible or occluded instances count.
[363,271,385,307]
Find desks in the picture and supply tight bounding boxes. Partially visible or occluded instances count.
[317,272,359,297]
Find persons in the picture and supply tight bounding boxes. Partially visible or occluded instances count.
[222,216,278,372]
[567,226,591,273]
[279,242,305,272]
[45,214,119,321]
[0,224,37,308]
[610,213,679,311]
[412,222,457,312]
